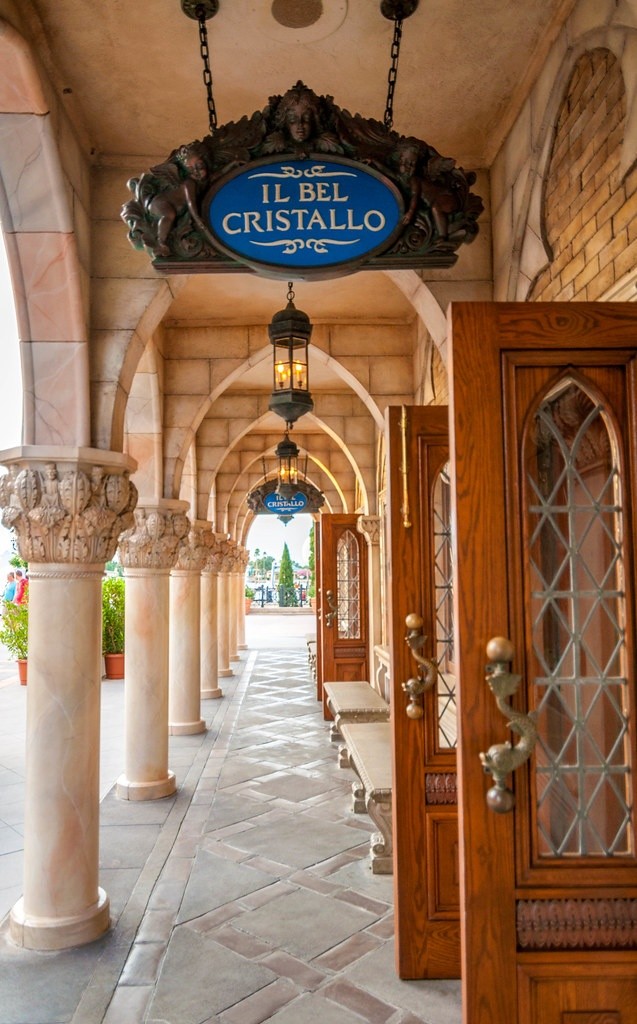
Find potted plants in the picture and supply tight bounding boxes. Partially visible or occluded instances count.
[307,586,316,614]
[245,587,255,615]
[102,577,125,679]
[0,583,29,685]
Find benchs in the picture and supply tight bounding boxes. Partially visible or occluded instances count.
[309,643,317,680]
[306,633,316,669]
[341,723,394,874]
[324,681,390,769]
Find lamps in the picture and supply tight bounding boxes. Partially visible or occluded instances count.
[275,423,300,502]
[269,282,313,429]
